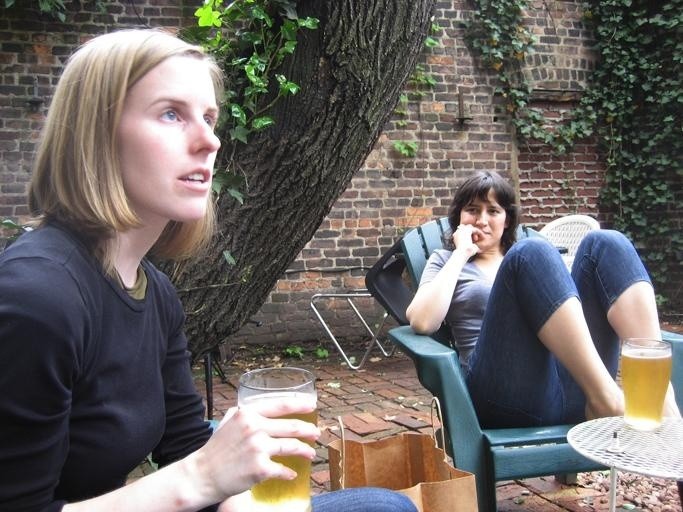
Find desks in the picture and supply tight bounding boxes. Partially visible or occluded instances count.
[567,414,683,512]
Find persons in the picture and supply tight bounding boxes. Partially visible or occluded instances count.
[0,27,419,512]
[405,170,682,430]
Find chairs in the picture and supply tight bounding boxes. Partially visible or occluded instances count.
[310,235,413,371]
[533,215,599,277]
[386,216,683,512]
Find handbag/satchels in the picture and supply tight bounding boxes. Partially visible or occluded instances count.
[327,397,479,512]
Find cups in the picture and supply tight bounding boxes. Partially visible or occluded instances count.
[620,338,674,435]
[236,366,319,512]
